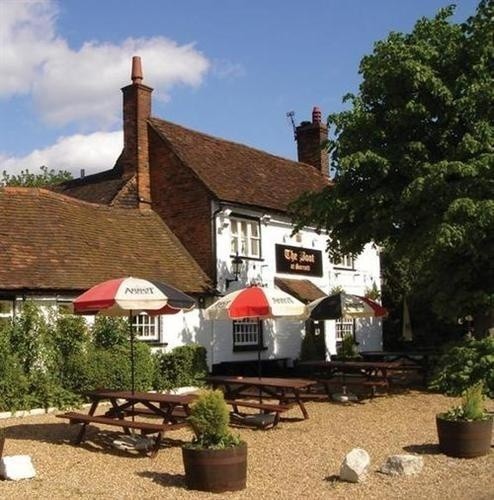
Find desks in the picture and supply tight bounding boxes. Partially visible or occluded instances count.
[195,375,316,428]
[76,390,202,458]
[318,357,429,396]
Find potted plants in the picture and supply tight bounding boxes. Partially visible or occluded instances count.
[436,379,492,458]
[182,389,247,493]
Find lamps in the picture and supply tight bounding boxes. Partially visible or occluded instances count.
[226,251,243,289]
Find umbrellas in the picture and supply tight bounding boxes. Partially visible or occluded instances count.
[201,285,305,425]
[72,277,196,451]
[306,292,388,402]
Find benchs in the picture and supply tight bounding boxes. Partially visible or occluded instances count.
[219,400,289,412]
[107,406,190,418]
[314,365,425,385]
[237,391,328,400]
[57,412,173,432]
